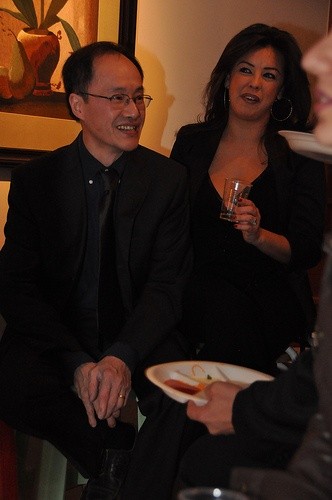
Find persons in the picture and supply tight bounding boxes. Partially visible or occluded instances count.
[0,42,222,500]
[171,24,332,471]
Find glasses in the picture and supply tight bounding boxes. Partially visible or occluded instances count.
[76,92,153,109]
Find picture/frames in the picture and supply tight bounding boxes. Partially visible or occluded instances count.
[0,0,138,163]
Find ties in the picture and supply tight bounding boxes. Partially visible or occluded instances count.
[97,170,126,346]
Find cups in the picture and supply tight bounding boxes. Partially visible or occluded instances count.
[219,176,253,222]
[177,484,254,500]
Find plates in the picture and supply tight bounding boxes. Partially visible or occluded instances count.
[277,130,332,164]
[144,359,277,411]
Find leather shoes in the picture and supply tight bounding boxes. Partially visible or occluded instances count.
[80,447,131,500]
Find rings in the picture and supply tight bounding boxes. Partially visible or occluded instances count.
[252,218,257,225]
[119,394,126,399]
